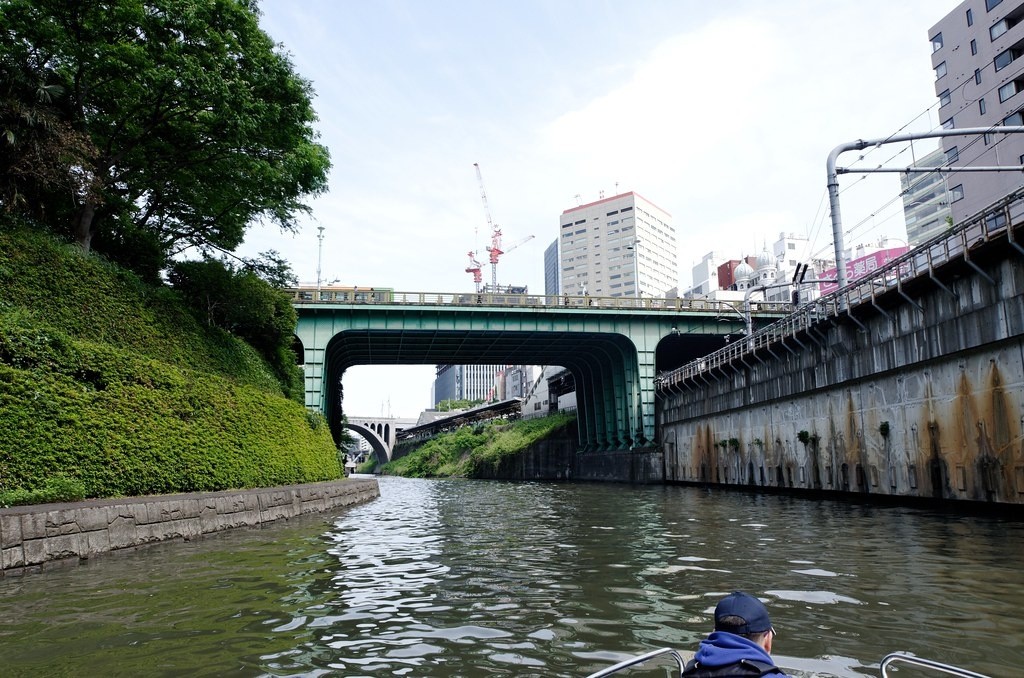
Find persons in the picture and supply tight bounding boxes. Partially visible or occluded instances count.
[757,300,762,310]
[705,296,709,303]
[689,296,692,309]
[680,297,684,308]
[587,293,592,306]
[564,293,570,306]
[354,285,359,300]
[477,291,483,304]
[370,288,375,301]
[682,590,794,678]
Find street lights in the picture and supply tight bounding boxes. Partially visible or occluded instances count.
[317,226,326,300]
[884,238,907,253]
[627,236,641,306]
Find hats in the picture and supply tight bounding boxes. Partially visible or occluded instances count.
[714,590,771,633]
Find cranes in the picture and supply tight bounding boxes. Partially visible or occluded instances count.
[465,226,491,293]
[473,163,536,293]
